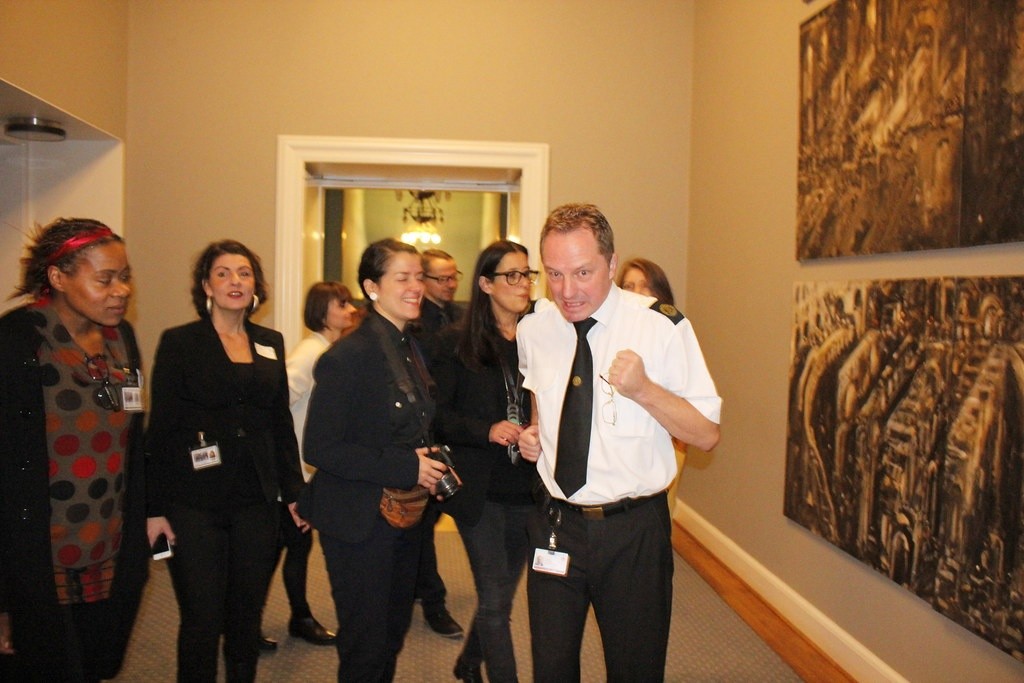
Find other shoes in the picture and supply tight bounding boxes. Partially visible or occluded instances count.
[453,657,482,683]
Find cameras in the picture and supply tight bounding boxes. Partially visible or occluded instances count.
[425,445,462,500]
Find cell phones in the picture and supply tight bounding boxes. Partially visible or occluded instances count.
[153,534,174,561]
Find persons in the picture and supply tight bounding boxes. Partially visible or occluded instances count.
[284,281,357,643]
[0,217,147,683]
[515,203,721,683]
[298,238,536,683]
[617,258,689,518]
[143,239,311,683]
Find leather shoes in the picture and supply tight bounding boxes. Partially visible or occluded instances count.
[422,611,465,637]
[288,614,336,645]
[260,635,278,651]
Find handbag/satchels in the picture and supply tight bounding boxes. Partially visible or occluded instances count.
[381,484,428,527]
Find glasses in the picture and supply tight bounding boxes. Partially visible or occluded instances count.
[423,270,464,285]
[86,354,122,413]
[488,270,539,286]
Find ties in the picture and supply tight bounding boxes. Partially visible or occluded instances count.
[553,317,598,499]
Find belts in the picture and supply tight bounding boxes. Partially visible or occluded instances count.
[539,490,668,520]
